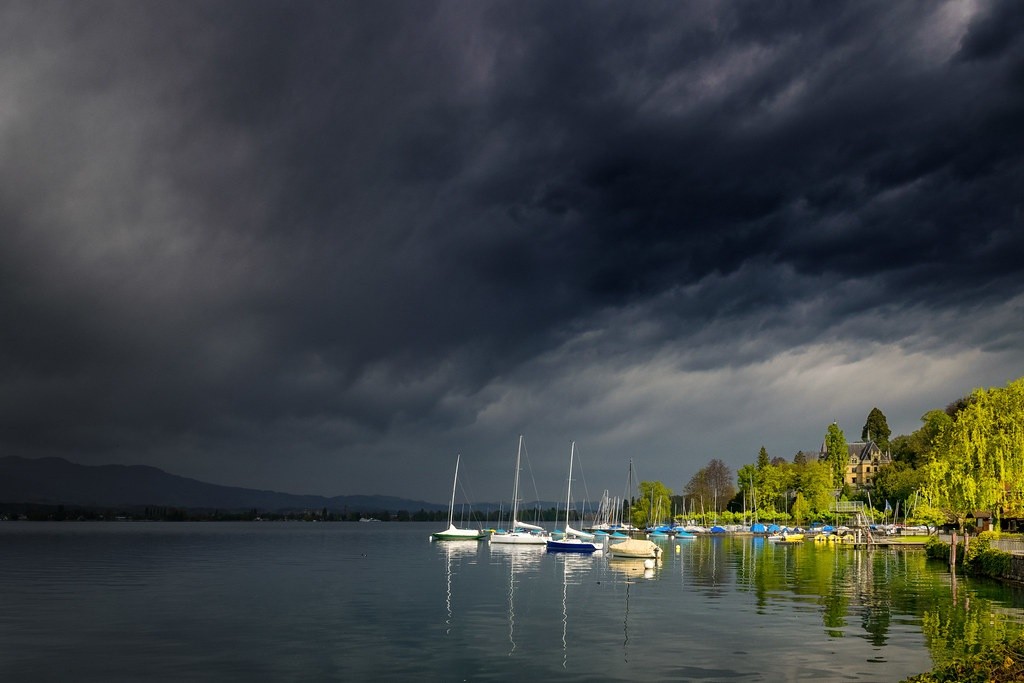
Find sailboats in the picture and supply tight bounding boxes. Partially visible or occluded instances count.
[607,455,664,559]
[431,454,489,541]
[489,432,554,545]
[580,472,946,539]
[544,439,605,554]
